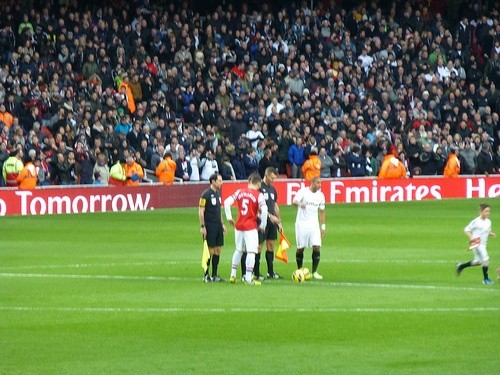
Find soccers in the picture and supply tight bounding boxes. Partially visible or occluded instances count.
[292,270,304,282]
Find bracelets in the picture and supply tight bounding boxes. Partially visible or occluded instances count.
[268,213,271,218]
[222,223,225,225]
[201,224,205,228]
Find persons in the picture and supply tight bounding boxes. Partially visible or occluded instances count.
[240,251,248,283]
[224,172,268,284]
[0,0,500,189]
[253,167,283,281]
[199,173,226,283]
[456,203,496,285]
[292,175,326,280]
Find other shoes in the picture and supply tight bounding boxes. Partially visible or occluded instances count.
[230,277,237,283]
[244,279,261,286]
[313,273,323,280]
[267,272,280,279]
[483,279,494,284]
[212,276,221,282]
[456,263,462,276]
[252,275,264,281]
[203,275,213,283]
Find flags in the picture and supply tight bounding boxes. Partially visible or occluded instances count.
[275,232,291,263]
[202,240,210,273]
